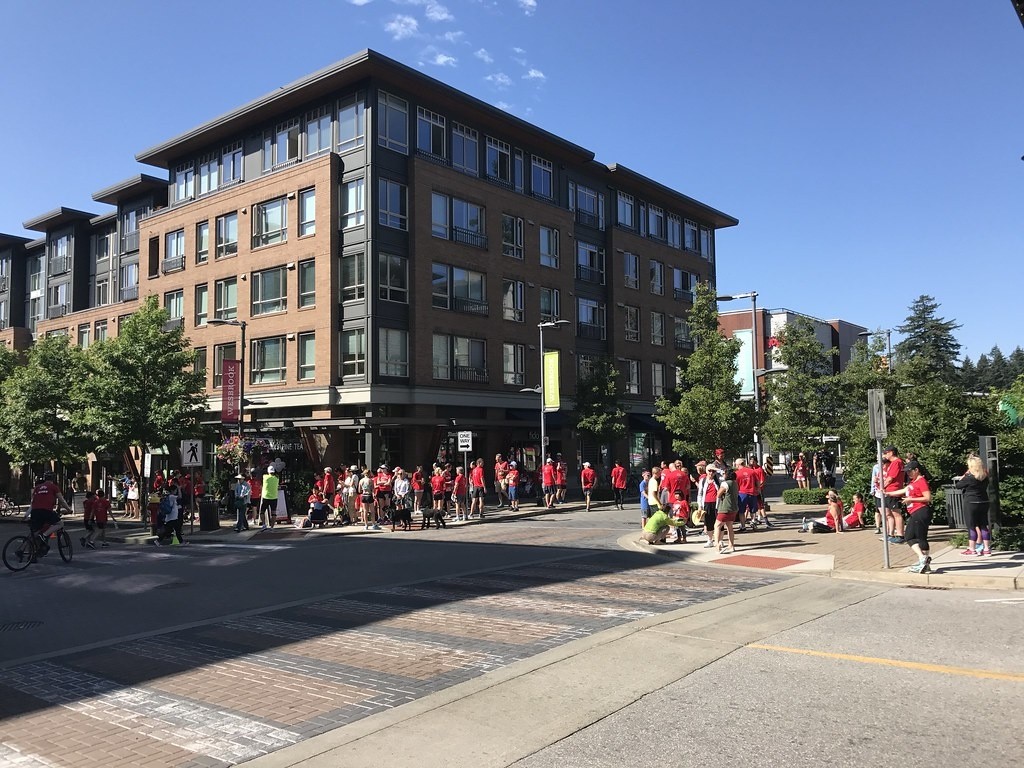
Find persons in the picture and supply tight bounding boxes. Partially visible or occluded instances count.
[785,446,905,534]
[259,465,279,532]
[611,459,627,510]
[79,491,99,548]
[246,471,262,525]
[580,462,598,512]
[638,449,772,553]
[71,471,87,493]
[506,460,519,512]
[882,460,933,574]
[494,454,510,508]
[233,473,251,532]
[307,457,487,532]
[87,489,116,549]
[29,471,73,557]
[107,469,204,548]
[903,452,921,486]
[536,457,567,509]
[955,456,992,557]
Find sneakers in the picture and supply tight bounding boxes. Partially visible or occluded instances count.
[891,534,898,542]
[910,564,931,573]
[919,554,931,573]
[960,547,978,557]
[978,548,992,557]
[893,536,905,543]
[650,517,775,554]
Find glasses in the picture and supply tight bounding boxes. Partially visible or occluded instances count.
[676,464,681,468]
[708,470,716,472]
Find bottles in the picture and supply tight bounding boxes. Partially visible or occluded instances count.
[114,519,119,531]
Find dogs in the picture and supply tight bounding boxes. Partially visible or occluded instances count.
[382,505,413,532]
[420,506,447,530]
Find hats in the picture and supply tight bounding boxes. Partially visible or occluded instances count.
[45,463,451,498]
[583,462,591,467]
[882,458,889,463]
[734,458,744,465]
[508,461,517,466]
[716,449,725,455]
[695,461,707,466]
[546,458,554,463]
[883,446,898,454]
[706,464,718,471]
[901,460,919,472]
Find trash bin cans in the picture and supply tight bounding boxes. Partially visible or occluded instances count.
[198,494,220,531]
[941,476,967,529]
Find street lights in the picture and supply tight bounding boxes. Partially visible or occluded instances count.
[206,318,267,521]
[520,320,571,494]
[860,329,916,414]
[716,290,789,466]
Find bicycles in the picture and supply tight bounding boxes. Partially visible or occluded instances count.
[2,510,73,572]
[622,469,640,497]
[842,464,850,484]
[0,492,21,517]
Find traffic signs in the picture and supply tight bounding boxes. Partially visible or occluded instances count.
[458,432,472,452]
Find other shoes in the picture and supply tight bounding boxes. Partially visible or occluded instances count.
[975,543,985,553]
[879,537,885,542]
[26,496,626,564]
[887,534,895,541]
[874,528,881,534]
[802,517,807,531]
